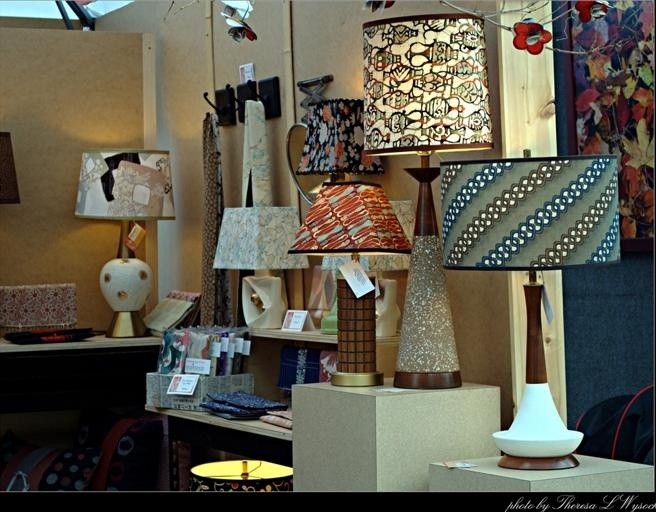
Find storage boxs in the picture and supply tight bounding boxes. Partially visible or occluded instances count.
[145,370,255,412]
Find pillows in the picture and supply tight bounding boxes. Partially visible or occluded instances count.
[1,407,165,492]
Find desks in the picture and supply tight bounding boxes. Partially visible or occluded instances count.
[0,332,163,413]
[143,398,290,491]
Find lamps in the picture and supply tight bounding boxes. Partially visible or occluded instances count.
[187,459,293,493]
[197,11,623,472]
[73,147,178,340]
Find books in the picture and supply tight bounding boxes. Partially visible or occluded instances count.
[140,297,196,339]
[164,288,203,330]
